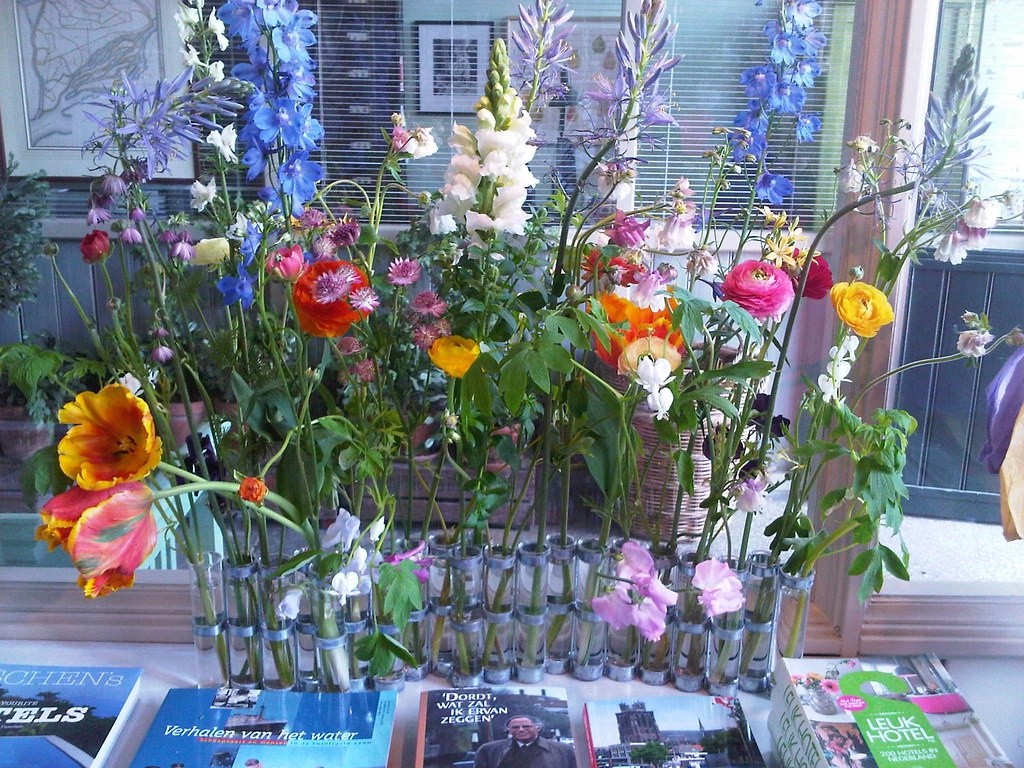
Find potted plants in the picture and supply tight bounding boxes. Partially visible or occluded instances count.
[0,343,75,462]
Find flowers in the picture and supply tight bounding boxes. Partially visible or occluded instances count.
[33,0,1023,696]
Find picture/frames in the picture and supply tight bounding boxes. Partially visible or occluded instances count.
[0,0,201,185]
